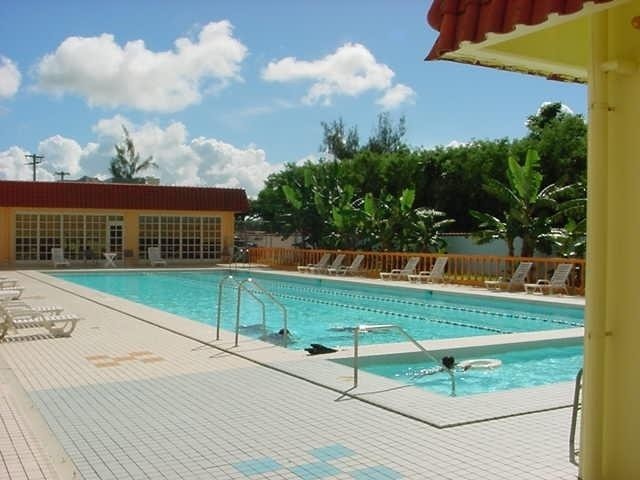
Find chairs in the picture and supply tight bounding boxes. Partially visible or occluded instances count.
[328,255,364,274]
[310,254,345,275]
[484,262,533,290]
[297,253,330,274]
[51,248,71,268]
[524,264,573,294]
[380,256,420,281]
[408,257,448,284]
[0,276,83,338]
[148,247,166,267]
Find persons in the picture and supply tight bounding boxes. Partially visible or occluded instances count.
[443,356,471,370]
